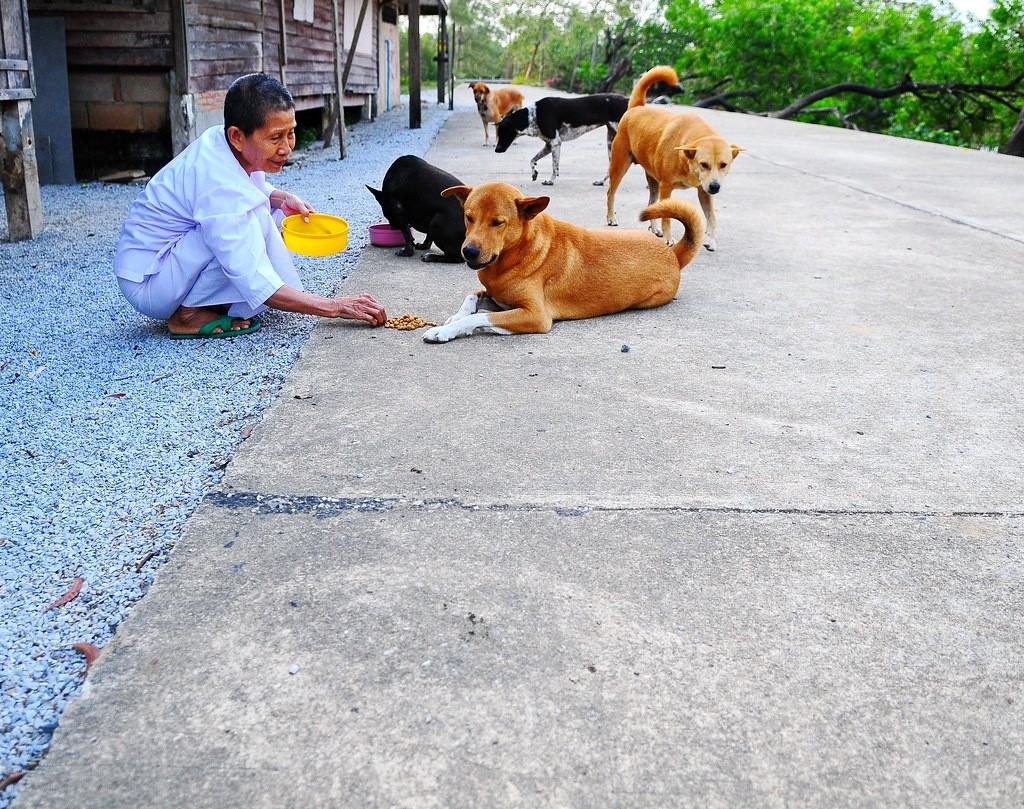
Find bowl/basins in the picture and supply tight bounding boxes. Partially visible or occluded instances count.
[280,212,350,257]
[369,224,411,246]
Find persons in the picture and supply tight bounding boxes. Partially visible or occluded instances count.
[113,72,387,340]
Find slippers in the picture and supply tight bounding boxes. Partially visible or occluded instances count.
[170,315,261,339]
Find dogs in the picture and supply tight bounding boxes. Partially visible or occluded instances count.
[469,81,525,145]
[364,154,467,263]
[607,65,747,252]
[423,182,704,345]
[490,92,630,186]
